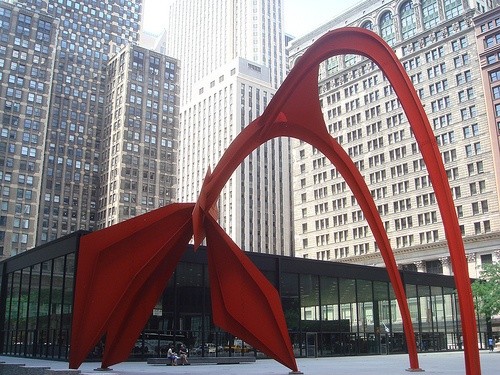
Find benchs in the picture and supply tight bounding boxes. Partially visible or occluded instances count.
[147,356,257,364]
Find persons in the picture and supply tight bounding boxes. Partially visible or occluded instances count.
[167,345,177,366]
[488,337,494,352]
[179,344,189,366]
